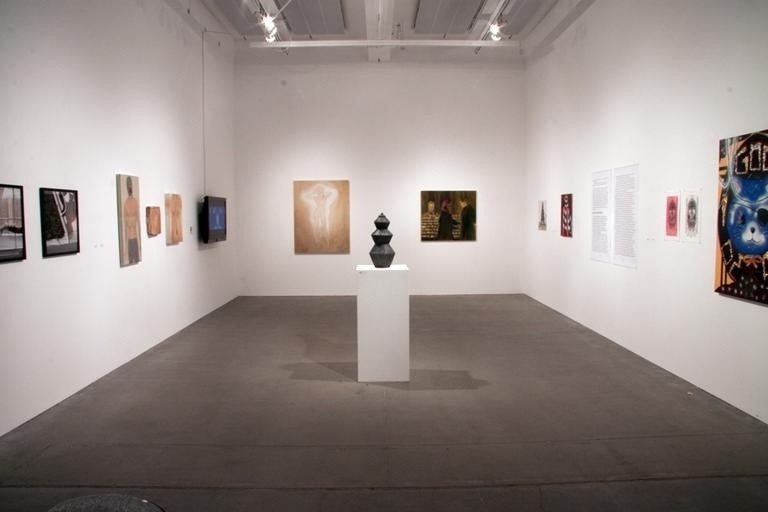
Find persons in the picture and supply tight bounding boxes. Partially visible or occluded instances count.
[459,197,476,239]
[436,198,460,240]
[124,176,140,266]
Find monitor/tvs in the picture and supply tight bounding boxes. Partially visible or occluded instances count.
[200,194,228,244]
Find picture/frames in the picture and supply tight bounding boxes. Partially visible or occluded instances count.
[39,188,80,258]
[0,184,26,264]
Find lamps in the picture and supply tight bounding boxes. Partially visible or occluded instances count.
[474,0,512,56]
[253,0,289,56]
